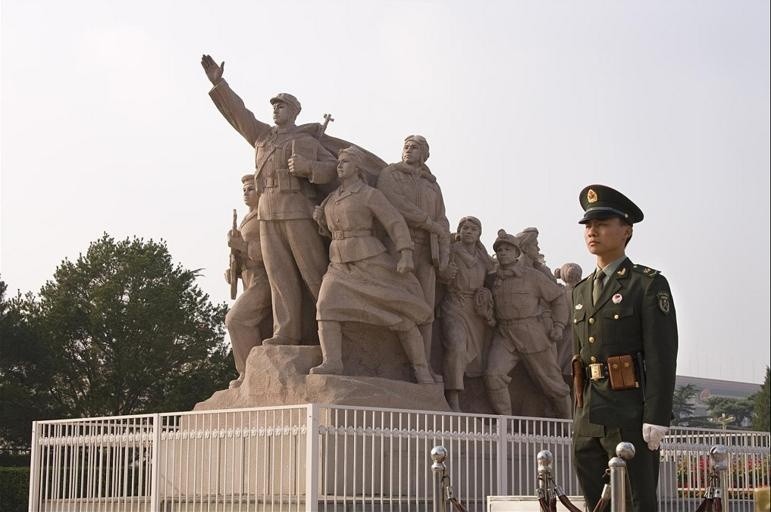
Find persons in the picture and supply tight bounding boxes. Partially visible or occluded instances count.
[570,185,678,511]
[475,226,586,438]
[439,215,494,413]
[223,174,275,388]
[378,134,449,385]
[309,146,435,385]
[200,54,338,344]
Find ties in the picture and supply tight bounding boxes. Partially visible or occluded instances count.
[593,270,605,306]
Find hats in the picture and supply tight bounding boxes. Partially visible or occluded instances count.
[578,185,643,226]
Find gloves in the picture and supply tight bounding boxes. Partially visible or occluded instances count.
[642,423,669,452]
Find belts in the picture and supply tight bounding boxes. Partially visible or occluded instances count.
[584,363,609,382]
